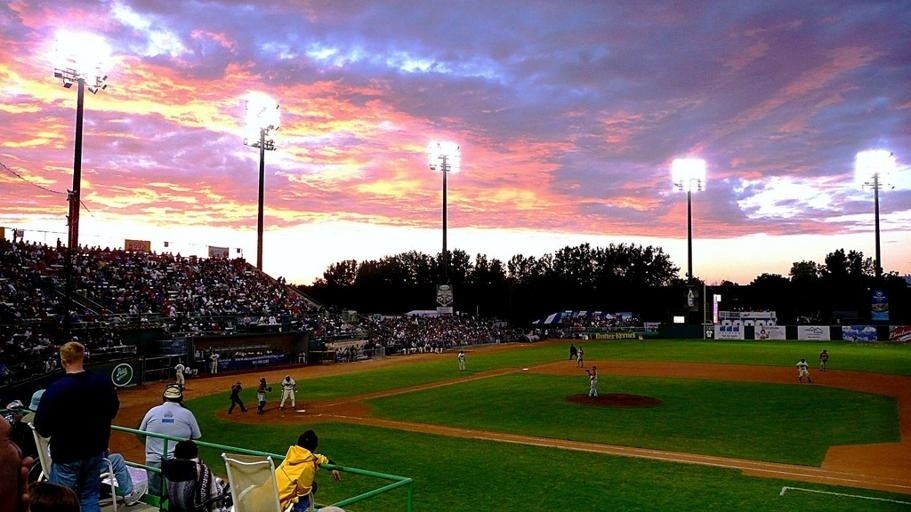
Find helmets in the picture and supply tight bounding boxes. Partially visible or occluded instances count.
[164,384,182,399]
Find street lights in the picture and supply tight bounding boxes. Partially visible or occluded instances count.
[242,93,283,273]
[671,158,707,277]
[52,31,115,248]
[429,139,461,254]
[853,148,901,275]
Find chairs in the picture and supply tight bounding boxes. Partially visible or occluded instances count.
[220,451,315,512]
[159,456,231,512]
[28,421,147,512]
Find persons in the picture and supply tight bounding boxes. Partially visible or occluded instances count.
[33,342,120,509]
[755,329,771,341]
[586,364,600,395]
[819,351,829,370]
[280,375,297,410]
[706,328,713,340]
[174,361,186,391]
[796,358,811,384]
[576,345,584,367]
[168,439,230,509]
[251,429,341,511]
[458,351,467,373]
[0,389,146,511]
[229,382,247,414]
[567,343,578,360]
[137,384,201,496]
[256,378,272,415]
[373,313,642,353]
[2,240,369,382]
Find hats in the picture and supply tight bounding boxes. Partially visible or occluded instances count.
[29,389,47,411]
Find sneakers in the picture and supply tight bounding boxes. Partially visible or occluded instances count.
[125,484,146,506]
